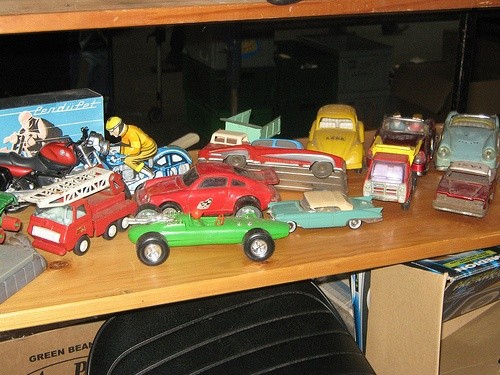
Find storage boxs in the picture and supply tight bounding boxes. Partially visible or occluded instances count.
[406,248,500,324]
[0,315,106,375]
[0,87,106,158]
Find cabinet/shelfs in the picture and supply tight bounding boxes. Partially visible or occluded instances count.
[0,0,500,334]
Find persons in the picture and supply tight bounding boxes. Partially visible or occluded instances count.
[389,113,424,133]
[106,117,157,179]
[3,111,62,151]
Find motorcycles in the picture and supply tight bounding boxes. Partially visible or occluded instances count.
[0,126,132,198]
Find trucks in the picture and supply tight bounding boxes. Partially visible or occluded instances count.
[197,110,280,164]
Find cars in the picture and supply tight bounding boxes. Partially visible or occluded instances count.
[26,130,429,266]
[433,113,500,172]
[431,160,499,218]
[306,104,366,174]
[362,112,441,176]
